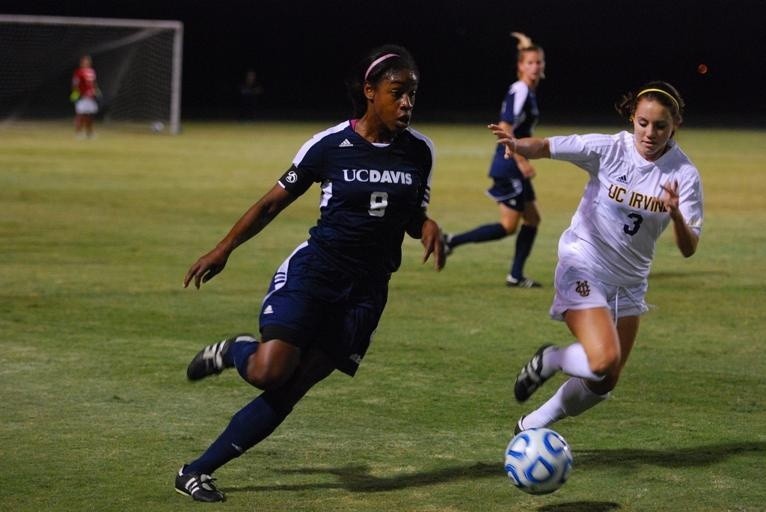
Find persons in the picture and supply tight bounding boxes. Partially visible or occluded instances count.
[173,43,446,504]
[442,33,545,289]
[484,81,704,439]
[69,55,100,137]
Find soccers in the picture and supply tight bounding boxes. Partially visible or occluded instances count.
[505,428,572,495]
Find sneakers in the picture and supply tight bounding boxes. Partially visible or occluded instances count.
[184,331,256,382]
[502,274,544,290]
[433,230,453,271]
[513,341,565,404]
[512,414,527,439]
[172,462,227,504]
